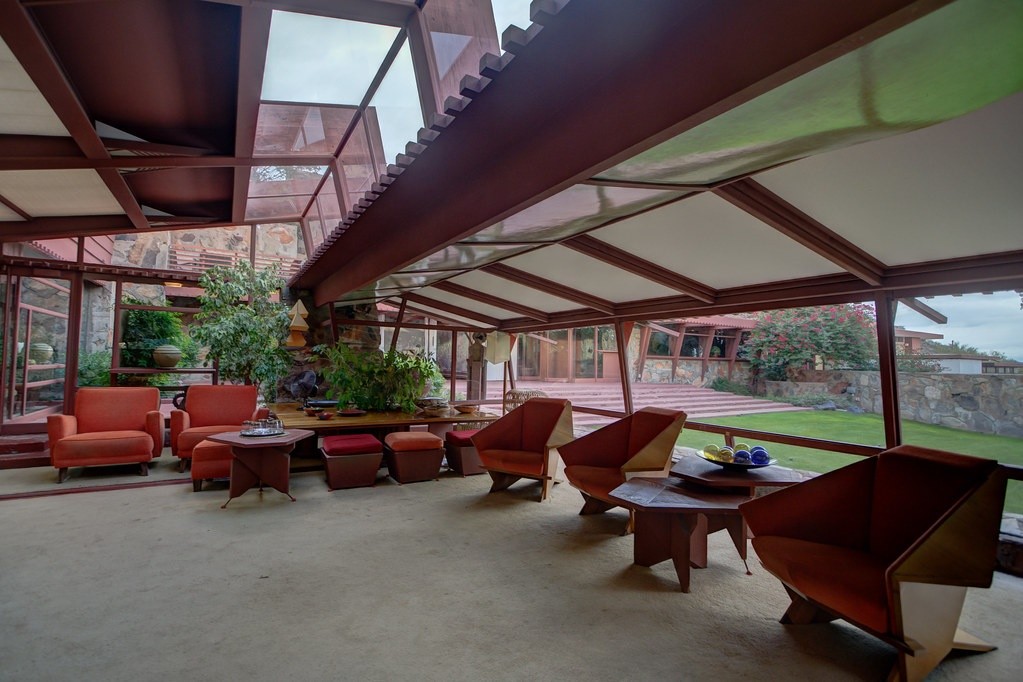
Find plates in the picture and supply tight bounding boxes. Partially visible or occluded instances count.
[696,449,776,473]
[337,410,366,416]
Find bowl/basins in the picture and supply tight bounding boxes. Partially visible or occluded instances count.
[423,406,450,416]
[303,408,324,416]
[413,397,448,410]
[454,405,479,413]
[315,413,334,420]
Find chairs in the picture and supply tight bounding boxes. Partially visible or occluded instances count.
[737,440,1012,682]
[556,404,688,538]
[469,395,574,503]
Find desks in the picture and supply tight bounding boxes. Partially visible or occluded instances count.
[607,452,820,593]
[205,428,315,511]
[268,401,499,473]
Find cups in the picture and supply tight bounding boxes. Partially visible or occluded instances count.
[241,418,283,434]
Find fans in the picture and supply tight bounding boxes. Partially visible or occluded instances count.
[290,370,321,411]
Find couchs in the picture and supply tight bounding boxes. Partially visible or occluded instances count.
[45,383,166,486]
[169,382,271,475]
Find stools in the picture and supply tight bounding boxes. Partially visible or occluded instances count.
[318,431,384,491]
[383,430,448,486]
[444,423,488,479]
[191,437,233,492]
